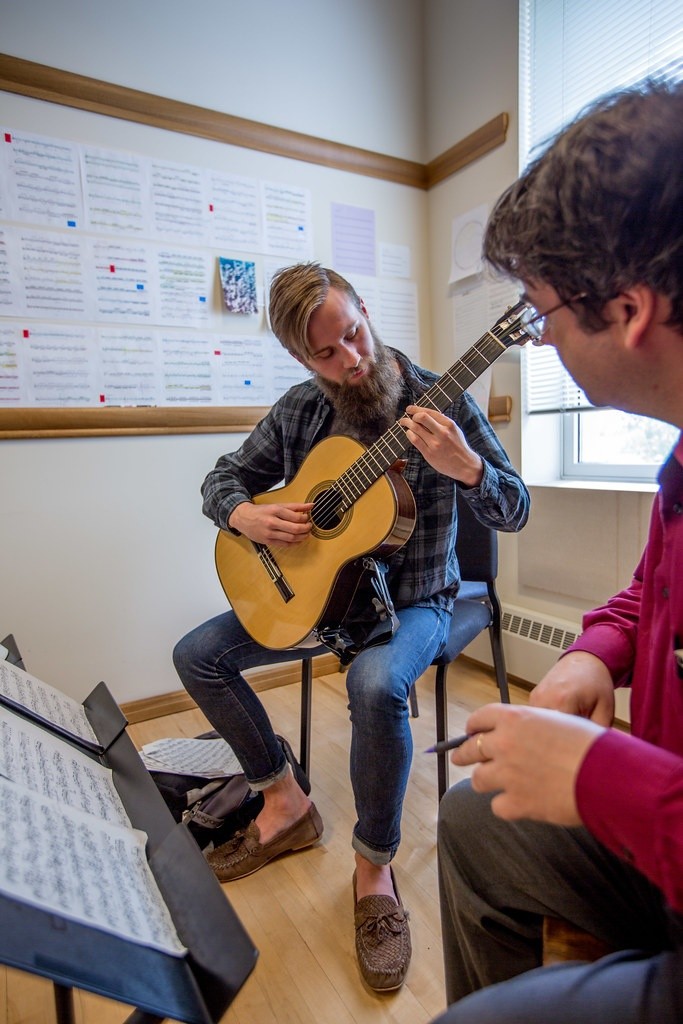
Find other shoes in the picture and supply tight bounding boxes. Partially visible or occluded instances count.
[205,800,324,881]
[352,862,412,991]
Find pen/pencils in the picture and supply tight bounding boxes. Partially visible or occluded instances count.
[105,404,157,408]
[425,734,473,754]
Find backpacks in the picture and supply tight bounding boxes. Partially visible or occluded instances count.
[148,729,310,850]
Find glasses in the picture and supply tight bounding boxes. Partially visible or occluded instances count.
[519,291,590,339]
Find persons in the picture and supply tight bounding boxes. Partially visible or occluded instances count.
[170,263,532,995]
[422,78,683,1024]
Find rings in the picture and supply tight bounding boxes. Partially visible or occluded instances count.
[477,733,488,763]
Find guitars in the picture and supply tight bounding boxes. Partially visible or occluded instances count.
[213,292,546,653]
